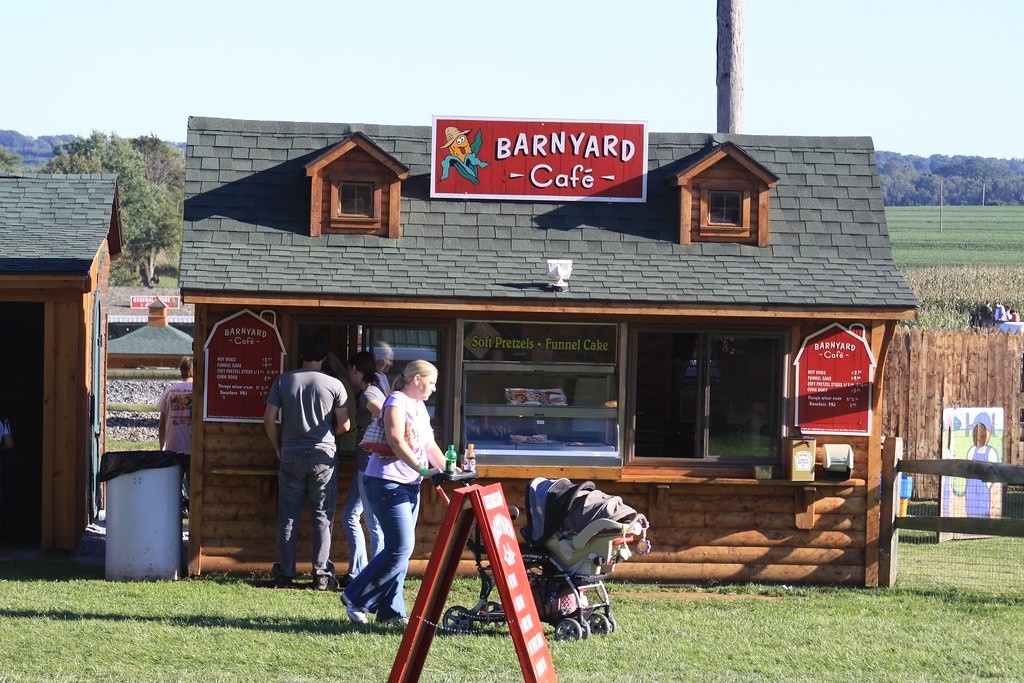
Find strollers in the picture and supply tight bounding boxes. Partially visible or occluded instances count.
[431,471,651,641]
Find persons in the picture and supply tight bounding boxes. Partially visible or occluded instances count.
[264,335,351,591]
[0,404,13,454]
[370,340,394,395]
[341,351,387,586]
[341,359,462,626]
[978,300,1018,324]
[158,355,194,479]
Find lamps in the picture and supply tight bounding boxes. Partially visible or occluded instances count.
[547,259,573,290]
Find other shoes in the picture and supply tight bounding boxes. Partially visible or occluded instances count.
[340,592,368,625]
[312,575,329,590]
[380,615,409,627]
[334,574,353,590]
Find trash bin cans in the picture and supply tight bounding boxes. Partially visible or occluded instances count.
[101,452,187,582]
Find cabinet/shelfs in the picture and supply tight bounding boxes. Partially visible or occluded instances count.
[455,359,626,466]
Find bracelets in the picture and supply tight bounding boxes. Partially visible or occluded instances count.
[419,462,427,477]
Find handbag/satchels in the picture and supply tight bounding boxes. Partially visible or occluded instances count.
[357,393,412,458]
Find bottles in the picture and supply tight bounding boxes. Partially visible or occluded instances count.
[464,444,476,472]
[445,445,457,472]
